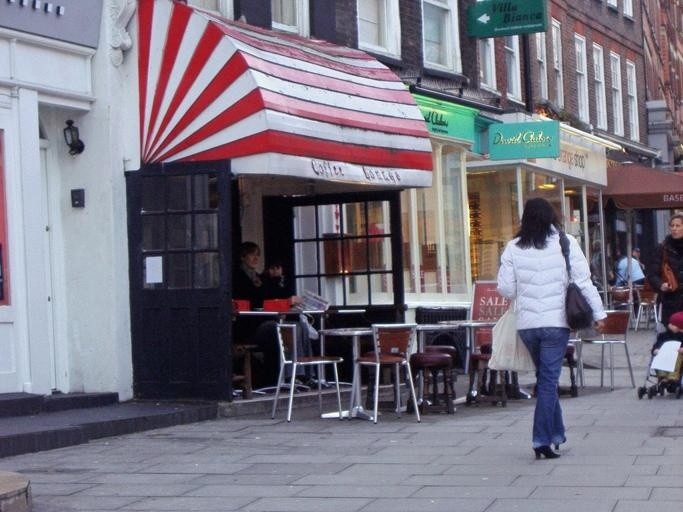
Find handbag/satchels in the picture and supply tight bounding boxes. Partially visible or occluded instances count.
[554,227,599,332]
[655,240,680,295]
[484,295,541,375]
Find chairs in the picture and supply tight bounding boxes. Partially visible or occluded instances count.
[573,309,636,393]
[349,323,422,425]
[234,340,262,400]
[594,280,663,334]
[271,323,345,422]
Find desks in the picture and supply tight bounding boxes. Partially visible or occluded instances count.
[439,319,529,401]
[316,323,459,421]
[235,309,366,393]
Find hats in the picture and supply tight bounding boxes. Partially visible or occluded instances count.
[665,309,683,329]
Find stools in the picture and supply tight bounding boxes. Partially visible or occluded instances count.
[530,342,576,399]
[425,344,458,401]
[406,354,458,415]
[462,353,510,409]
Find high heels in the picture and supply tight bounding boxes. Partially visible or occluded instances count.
[553,435,567,450]
[533,441,561,460]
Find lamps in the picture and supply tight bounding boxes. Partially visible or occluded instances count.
[62,119,86,155]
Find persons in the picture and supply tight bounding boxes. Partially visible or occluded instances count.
[262,258,297,297]
[592,213,683,393]
[497,197,608,459]
[232,241,330,391]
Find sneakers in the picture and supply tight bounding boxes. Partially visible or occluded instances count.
[284,378,309,392]
[657,378,679,393]
[302,376,332,390]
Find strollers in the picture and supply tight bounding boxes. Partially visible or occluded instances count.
[635,283,683,399]
